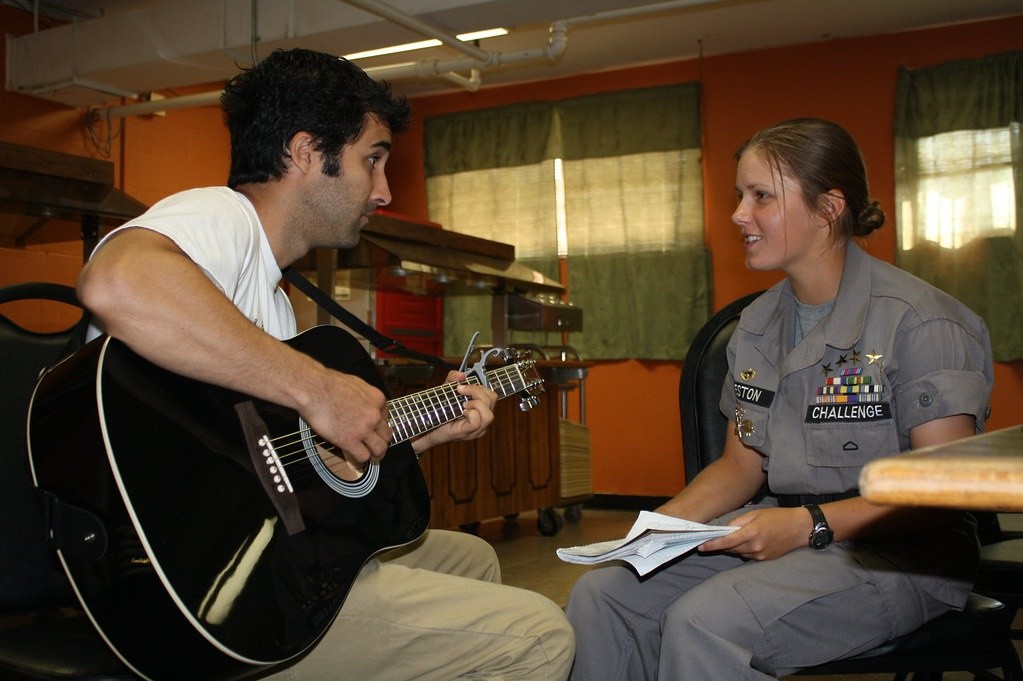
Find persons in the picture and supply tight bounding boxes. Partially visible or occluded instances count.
[76,47,577,681]
[564,118,993,681]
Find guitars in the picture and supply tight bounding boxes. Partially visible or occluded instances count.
[26,326,548,680]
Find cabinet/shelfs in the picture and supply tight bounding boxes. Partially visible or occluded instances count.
[378,358,561,529]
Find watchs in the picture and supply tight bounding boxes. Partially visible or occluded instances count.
[801,504,834,550]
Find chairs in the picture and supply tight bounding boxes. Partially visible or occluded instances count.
[1,280,125,681]
[678,287,1023,681]
[976,511,1023,630]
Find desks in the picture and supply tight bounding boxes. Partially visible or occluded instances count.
[859,424,1023,513]
